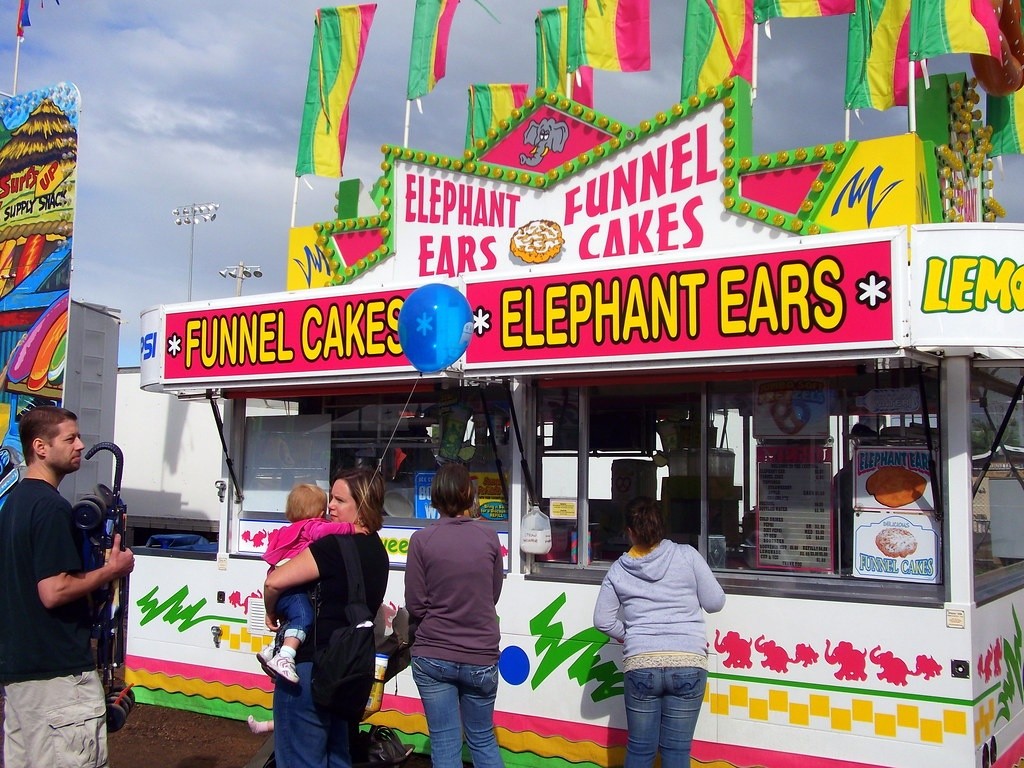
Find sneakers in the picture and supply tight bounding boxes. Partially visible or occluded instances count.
[256,647,276,678]
[266,654,299,684]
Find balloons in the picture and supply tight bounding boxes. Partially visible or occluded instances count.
[398,284,472,375]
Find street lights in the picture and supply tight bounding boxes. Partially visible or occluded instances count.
[219,261,264,296]
[171,201,220,302]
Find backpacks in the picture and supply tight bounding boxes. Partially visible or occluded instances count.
[311,533,375,722]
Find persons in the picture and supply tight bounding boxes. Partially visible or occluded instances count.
[593,494,725,768]
[264,469,390,767]
[403,460,511,768]
[0,407,137,767]
[256,481,370,686]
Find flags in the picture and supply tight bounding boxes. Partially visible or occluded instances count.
[16,0,31,41]
[294,1,1024,185]
